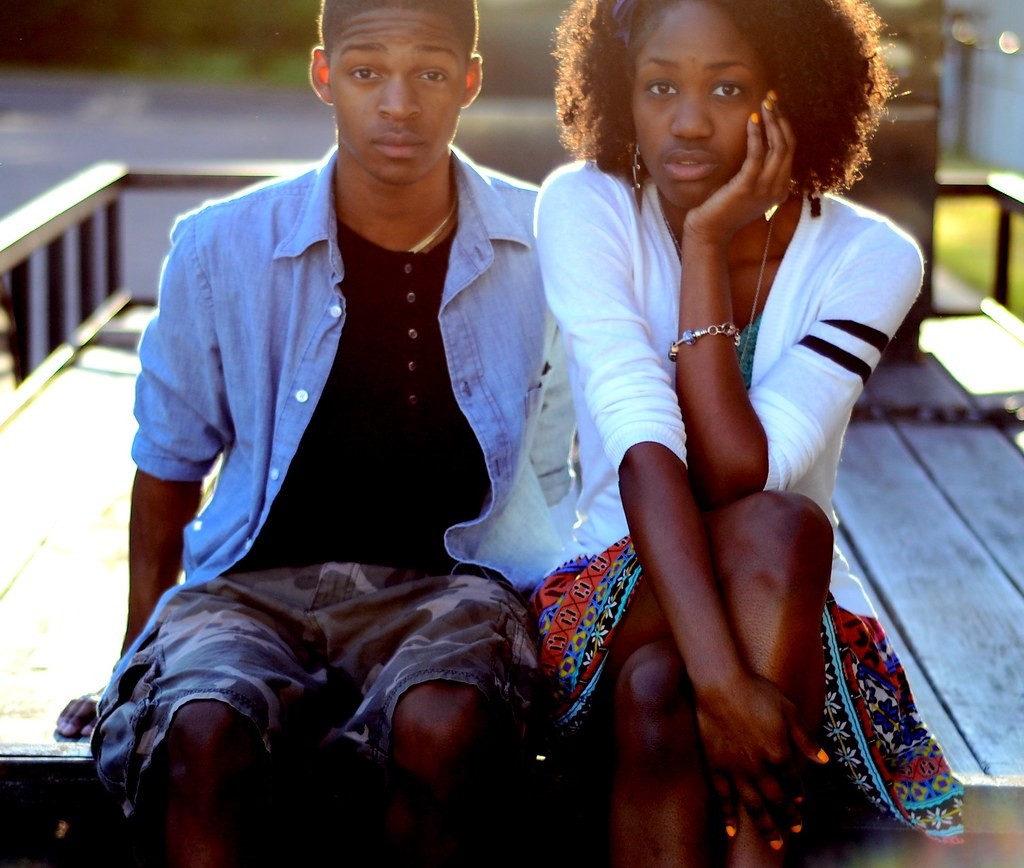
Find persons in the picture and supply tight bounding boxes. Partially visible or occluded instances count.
[56,1,580,868]
[474,1,965,868]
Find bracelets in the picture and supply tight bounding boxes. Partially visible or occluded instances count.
[668,324,741,362]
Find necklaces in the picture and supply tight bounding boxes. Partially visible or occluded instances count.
[409,194,455,252]
[659,203,775,363]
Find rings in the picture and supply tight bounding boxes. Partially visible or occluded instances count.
[787,180,799,193]
